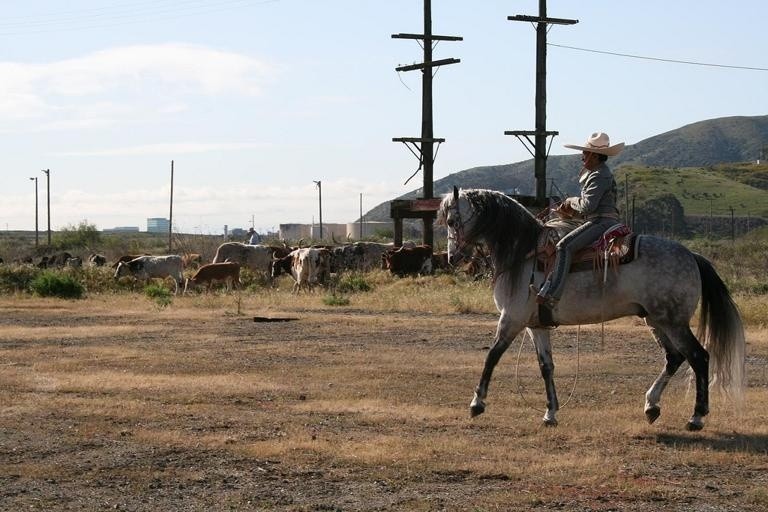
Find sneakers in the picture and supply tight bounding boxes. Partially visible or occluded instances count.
[530,283,557,310]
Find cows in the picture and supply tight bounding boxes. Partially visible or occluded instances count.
[212,232,454,296]
[111,253,202,296]
[88,253,106,266]
[184,262,240,295]
[37,252,82,269]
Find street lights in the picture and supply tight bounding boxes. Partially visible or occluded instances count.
[40,167,51,246]
[248,214,255,227]
[29,177,38,248]
[311,179,323,241]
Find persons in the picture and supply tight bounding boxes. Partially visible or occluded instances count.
[247,227,258,245]
[529,131,625,310]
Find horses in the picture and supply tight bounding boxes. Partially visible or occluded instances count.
[432,184,748,432]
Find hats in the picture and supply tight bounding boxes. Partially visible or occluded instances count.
[564,132,625,157]
